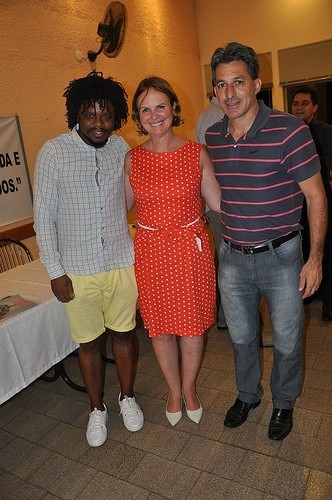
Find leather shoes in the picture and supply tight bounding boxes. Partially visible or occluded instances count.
[224,398,262,428]
[320,308,332,325]
[304,295,316,305]
[268,407,291,440]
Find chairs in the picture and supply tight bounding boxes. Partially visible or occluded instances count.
[0,238,32,275]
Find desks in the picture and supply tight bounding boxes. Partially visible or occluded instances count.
[0,257,115,405]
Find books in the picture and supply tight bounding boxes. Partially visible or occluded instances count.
[0,294,37,322]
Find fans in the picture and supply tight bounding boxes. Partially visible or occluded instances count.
[88,2,127,62]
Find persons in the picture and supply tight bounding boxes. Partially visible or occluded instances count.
[291,88,332,322]
[33,75,144,448]
[123,76,221,426]
[196,80,228,329]
[204,41,328,441]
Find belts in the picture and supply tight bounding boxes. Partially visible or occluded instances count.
[222,230,299,254]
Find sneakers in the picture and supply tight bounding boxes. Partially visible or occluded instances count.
[118,391,144,432]
[85,402,108,447]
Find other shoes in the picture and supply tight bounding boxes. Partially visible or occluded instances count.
[166,395,183,427]
[217,326,227,330]
[182,390,203,424]
